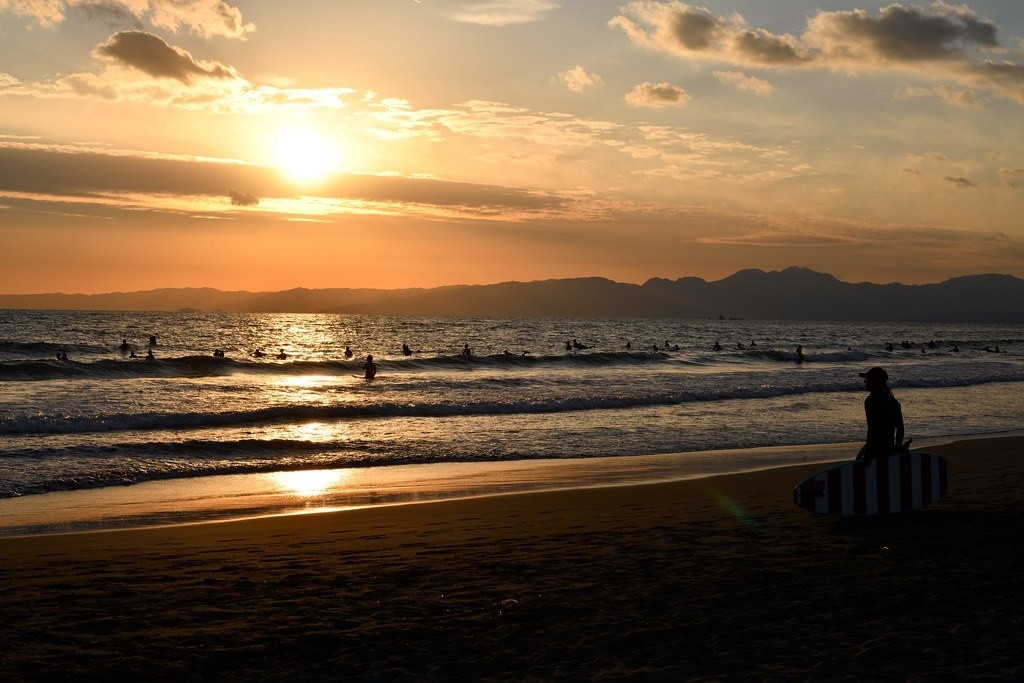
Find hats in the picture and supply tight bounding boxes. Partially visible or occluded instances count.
[859,367,888,383]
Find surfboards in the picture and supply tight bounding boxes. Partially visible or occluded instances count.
[792,451,949,516]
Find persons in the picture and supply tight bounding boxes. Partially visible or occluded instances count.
[56,352,70,363]
[119,334,157,359]
[857,366,905,461]
[565,338,758,351]
[344,346,353,359]
[276,348,287,360]
[361,354,377,383]
[253,349,262,358]
[462,344,471,356]
[847,339,1000,354]
[795,345,804,364]
[503,349,529,359]
[213,349,225,358]
